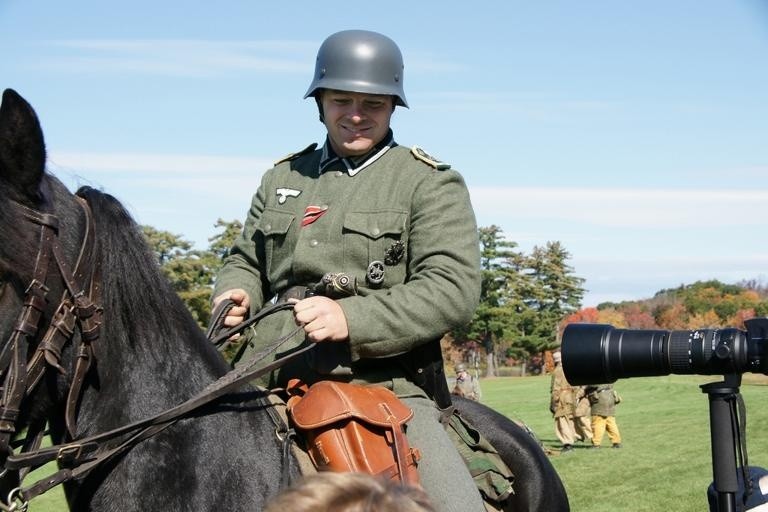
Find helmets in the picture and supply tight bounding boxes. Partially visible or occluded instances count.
[302,29,412,110]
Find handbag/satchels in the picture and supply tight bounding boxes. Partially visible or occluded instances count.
[283,374,423,494]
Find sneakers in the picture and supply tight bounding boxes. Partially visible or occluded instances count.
[560,443,621,454]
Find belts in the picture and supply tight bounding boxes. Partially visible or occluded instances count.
[594,387,611,393]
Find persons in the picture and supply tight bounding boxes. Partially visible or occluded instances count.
[209,30,487,512]
[550,353,578,451]
[572,385,593,443]
[584,382,621,449]
[514,420,559,455]
[452,365,481,402]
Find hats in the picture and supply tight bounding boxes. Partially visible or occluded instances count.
[454,364,467,374]
[552,351,561,362]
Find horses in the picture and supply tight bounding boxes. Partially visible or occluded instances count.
[0,87,571,512]
